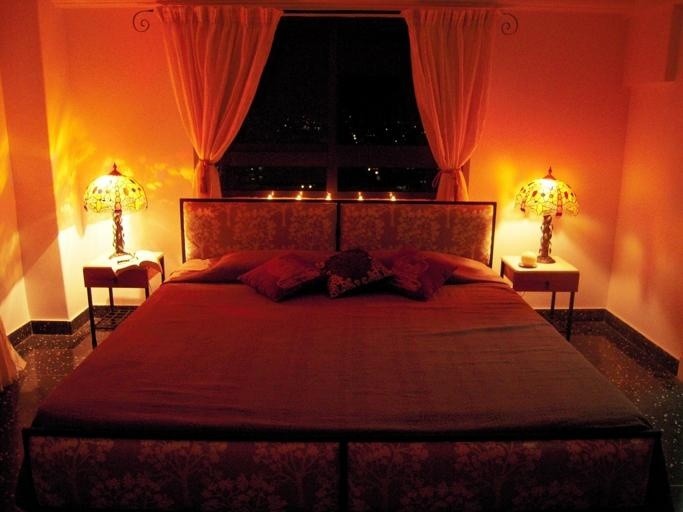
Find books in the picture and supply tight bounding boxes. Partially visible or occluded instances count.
[110,250,162,278]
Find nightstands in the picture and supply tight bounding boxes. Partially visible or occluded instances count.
[501,254,579,342]
[83,251,164,350]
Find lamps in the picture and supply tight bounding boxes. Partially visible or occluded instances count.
[82,161,148,263]
[514,166,581,264]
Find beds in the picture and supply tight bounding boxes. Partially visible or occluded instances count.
[18,199,668,512]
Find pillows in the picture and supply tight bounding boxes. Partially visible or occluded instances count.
[237,250,325,303]
[322,248,393,299]
[164,247,338,286]
[368,249,460,302]
[405,249,507,285]
